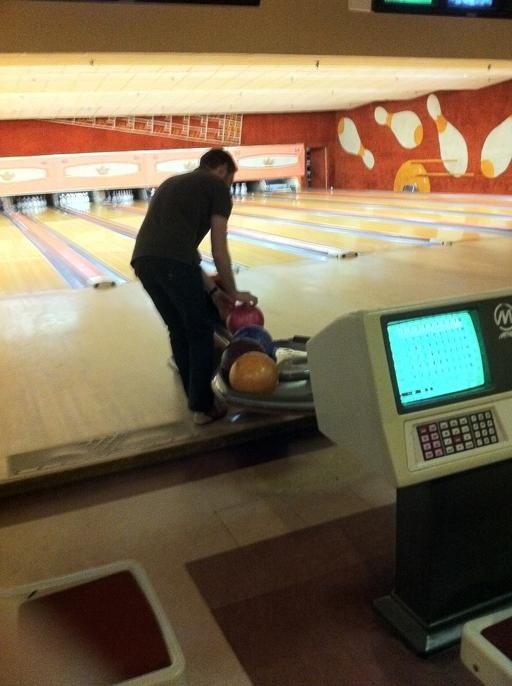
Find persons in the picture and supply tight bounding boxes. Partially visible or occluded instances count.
[130,148,259,426]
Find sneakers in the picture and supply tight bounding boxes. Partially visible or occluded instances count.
[189,400,229,426]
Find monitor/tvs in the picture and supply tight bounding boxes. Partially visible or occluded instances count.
[380,301,501,416]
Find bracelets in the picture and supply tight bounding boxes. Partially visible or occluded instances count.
[207,286,217,298]
